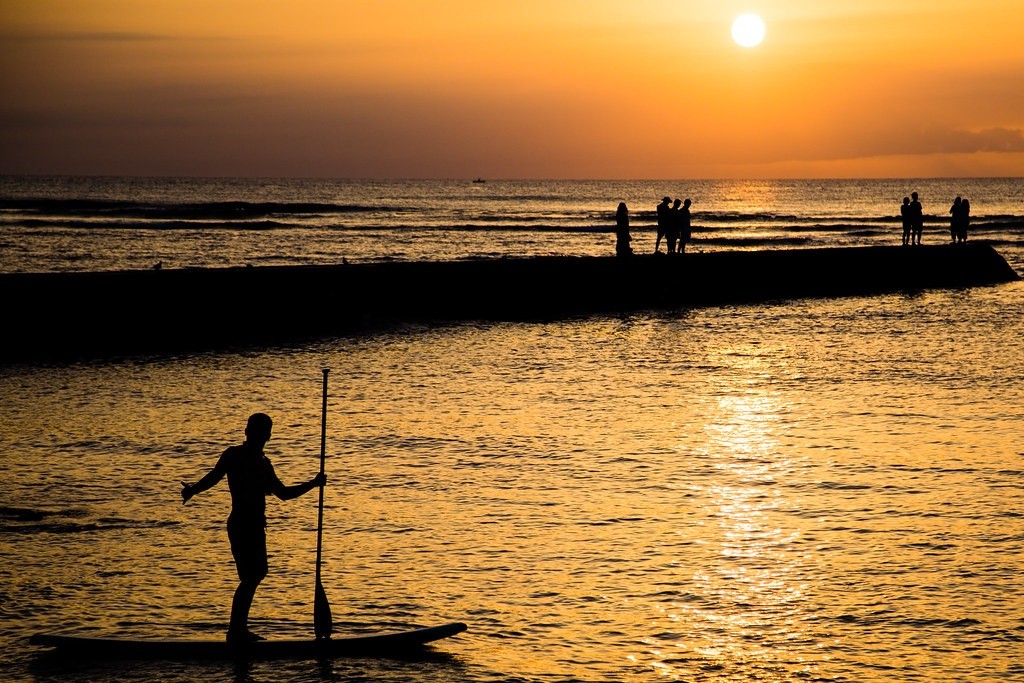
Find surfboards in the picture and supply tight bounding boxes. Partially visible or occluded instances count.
[27,621,470,650]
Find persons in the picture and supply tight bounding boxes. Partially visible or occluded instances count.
[654,197,692,253]
[901,192,923,245]
[616,202,633,254]
[180,413,327,640]
[949,197,970,243]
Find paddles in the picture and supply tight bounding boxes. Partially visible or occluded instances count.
[313,363,336,641]
